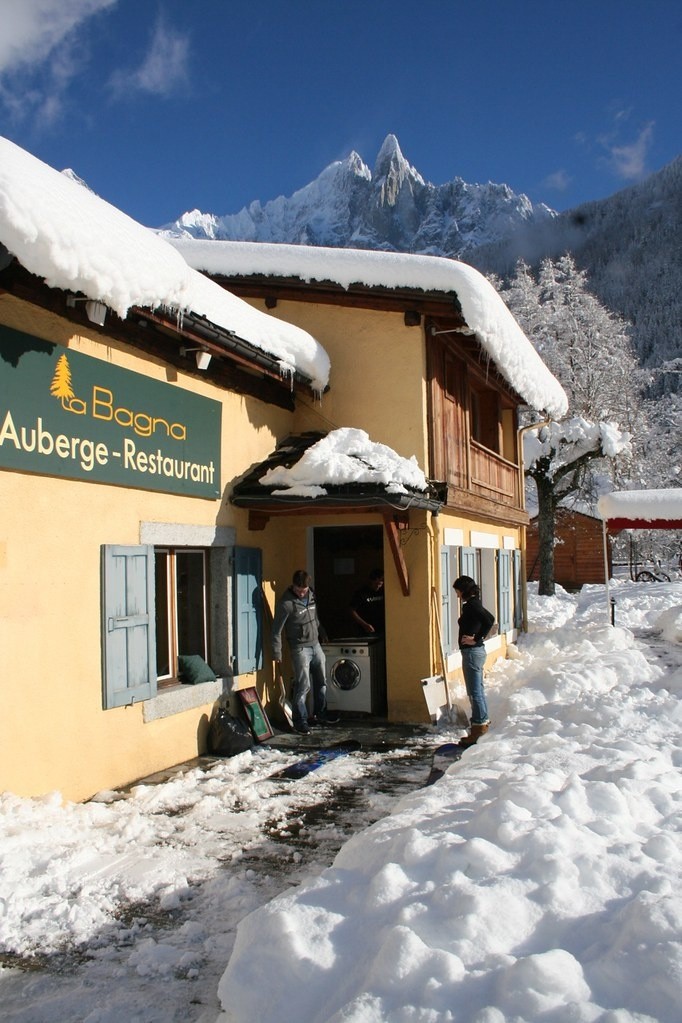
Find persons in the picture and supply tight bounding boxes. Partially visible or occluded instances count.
[270,569,341,735]
[345,569,385,633]
[453,575,495,745]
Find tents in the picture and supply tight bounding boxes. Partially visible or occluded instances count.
[599,489,682,624]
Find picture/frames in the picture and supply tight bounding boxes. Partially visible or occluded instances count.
[234,686,275,743]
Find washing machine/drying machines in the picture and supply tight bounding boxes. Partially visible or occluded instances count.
[321,635,386,714]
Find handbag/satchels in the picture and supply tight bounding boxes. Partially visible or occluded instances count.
[207,706,253,757]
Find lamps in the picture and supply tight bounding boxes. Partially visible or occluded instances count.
[66,295,107,327]
[181,345,212,370]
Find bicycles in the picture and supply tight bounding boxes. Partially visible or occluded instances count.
[636,553,671,584]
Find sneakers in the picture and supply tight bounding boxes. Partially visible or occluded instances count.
[293,720,310,735]
[314,711,340,724]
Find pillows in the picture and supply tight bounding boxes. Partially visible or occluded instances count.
[178,655,217,685]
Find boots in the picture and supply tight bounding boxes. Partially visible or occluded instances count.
[459,719,492,748]
